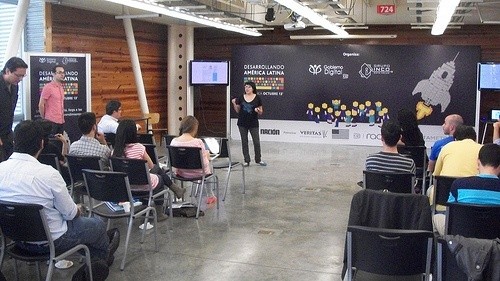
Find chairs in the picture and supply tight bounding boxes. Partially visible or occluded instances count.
[346,146,500,281]
[201,137,246,201]
[110,157,172,237]
[0,200,94,281]
[82,170,158,269]
[39,133,171,207]
[164,146,220,220]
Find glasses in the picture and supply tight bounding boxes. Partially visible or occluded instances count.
[13,72,27,78]
[119,108,123,112]
[56,71,66,74]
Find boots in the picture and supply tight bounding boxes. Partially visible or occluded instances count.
[156,204,168,221]
[169,183,186,199]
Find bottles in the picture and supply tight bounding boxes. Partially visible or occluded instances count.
[213,66,217,81]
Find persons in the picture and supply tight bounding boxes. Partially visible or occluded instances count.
[169,115,217,204]
[492,114,500,146]
[426,126,484,215]
[395,109,428,168]
[96,100,142,136]
[69,112,124,213]
[364,118,417,195]
[110,119,187,222]
[0,120,121,267]
[446,143,500,207]
[428,114,464,174]
[0,57,28,161]
[232,81,267,167]
[36,119,69,171]
[40,63,66,164]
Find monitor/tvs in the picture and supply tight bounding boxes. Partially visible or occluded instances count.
[189,60,230,85]
[477,61,500,91]
[489,108,500,121]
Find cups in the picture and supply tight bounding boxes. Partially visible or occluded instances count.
[124,202,130,212]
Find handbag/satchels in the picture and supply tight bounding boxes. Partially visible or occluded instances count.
[163,203,204,218]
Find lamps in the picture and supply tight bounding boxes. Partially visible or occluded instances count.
[265,8,276,22]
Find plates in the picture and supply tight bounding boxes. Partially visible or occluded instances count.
[139,224,153,230]
[55,260,73,268]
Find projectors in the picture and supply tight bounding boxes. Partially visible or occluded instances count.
[284,21,306,31]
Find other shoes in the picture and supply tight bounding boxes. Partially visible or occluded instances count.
[207,197,216,204]
[257,160,267,167]
[242,162,251,167]
[106,227,120,266]
